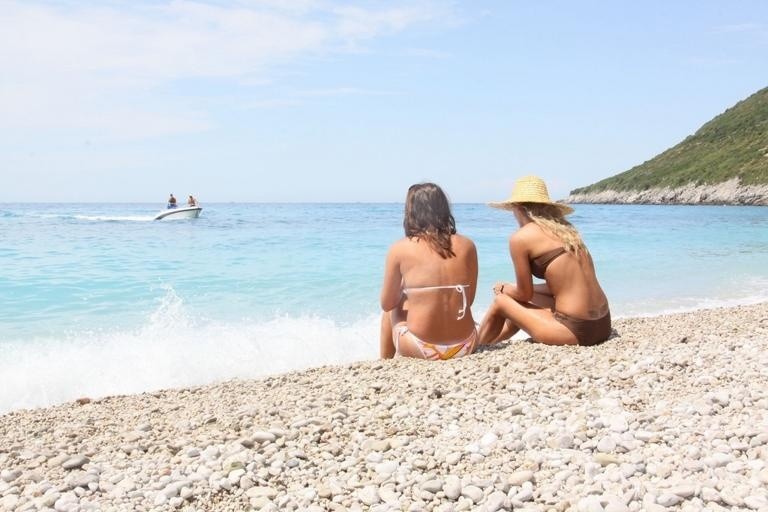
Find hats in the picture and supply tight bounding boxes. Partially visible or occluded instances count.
[488,176,574,216]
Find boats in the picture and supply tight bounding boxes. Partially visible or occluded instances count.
[154,206,202,220]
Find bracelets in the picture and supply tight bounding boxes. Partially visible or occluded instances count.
[498,284,505,294]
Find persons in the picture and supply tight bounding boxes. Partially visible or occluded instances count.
[375,181,484,363]
[165,193,178,210]
[185,193,198,207]
[471,171,615,348]
